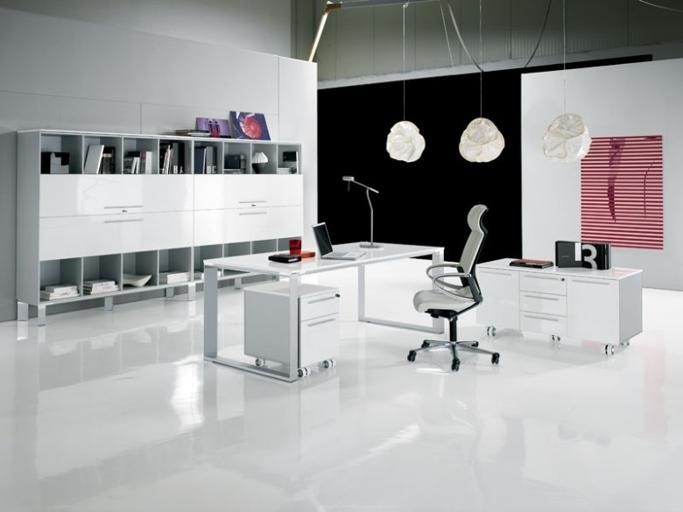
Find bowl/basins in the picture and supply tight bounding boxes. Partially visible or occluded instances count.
[252,163,268,174]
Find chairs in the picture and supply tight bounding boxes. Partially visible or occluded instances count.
[407,205,499,372]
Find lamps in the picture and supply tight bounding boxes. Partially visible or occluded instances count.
[339,175,384,250]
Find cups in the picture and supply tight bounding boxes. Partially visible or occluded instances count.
[288,236,302,256]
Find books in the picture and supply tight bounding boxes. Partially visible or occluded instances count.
[269,254,301,263]
[283,151,299,174]
[85,142,217,174]
[40,271,221,301]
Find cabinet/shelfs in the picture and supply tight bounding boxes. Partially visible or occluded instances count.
[474,257,643,354]
[18,130,194,324]
[195,137,302,289]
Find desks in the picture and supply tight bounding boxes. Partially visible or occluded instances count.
[203,240,445,383]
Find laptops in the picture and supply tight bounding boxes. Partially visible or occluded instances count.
[312,222,367,260]
